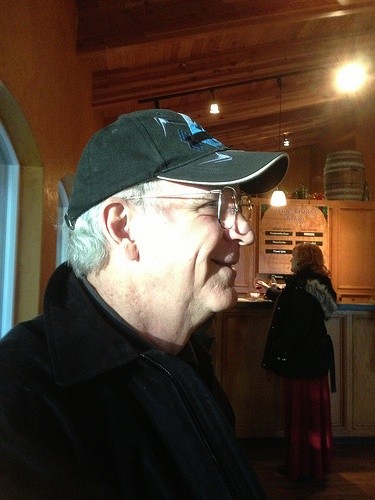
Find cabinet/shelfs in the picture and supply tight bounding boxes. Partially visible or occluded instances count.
[230,197,375,304]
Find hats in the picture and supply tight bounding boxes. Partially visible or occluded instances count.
[64,108,289,231]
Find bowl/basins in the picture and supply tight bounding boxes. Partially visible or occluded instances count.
[250,292,260,300]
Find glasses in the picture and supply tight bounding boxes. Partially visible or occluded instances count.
[125,185,252,229]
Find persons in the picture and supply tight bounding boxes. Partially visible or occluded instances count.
[0,108,289,500]
[253,243,337,493]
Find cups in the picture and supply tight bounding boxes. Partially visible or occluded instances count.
[299,187,308,199]
[293,191,300,199]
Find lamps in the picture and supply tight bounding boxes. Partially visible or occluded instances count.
[269,77,287,208]
[210,88,220,115]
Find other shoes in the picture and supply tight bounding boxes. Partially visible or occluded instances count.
[285,476,311,487]
[278,465,292,477]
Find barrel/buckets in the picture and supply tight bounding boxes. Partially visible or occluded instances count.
[323,150,368,200]
